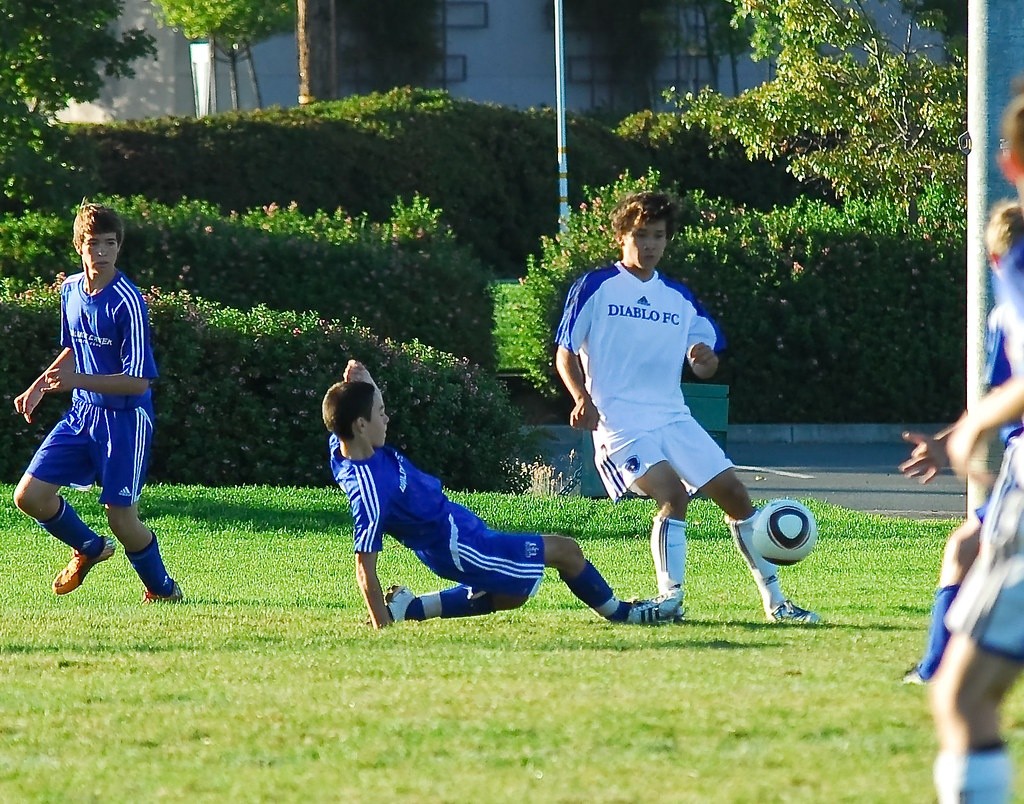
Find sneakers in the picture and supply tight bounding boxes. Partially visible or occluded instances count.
[628,589,684,625]
[52,536,115,595]
[769,600,820,625]
[384,585,415,622]
[898,663,925,686]
[143,582,182,604]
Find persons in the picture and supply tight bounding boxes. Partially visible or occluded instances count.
[555,192,817,623]
[13,195,185,603]
[923,86,1024,804]
[900,195,1024,685]
[321,360,684,630]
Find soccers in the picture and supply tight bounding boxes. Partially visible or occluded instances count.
[752,499,818,567]
